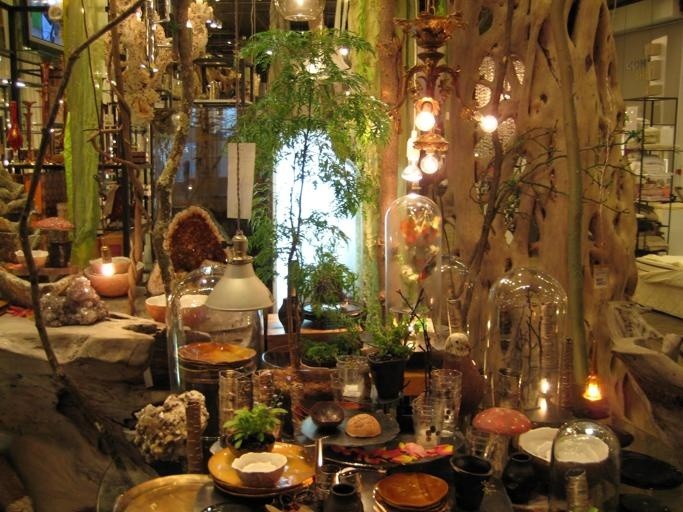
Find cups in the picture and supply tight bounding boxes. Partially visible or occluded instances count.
[449,455,493,509]
[317,464,339,485]
[216,354,370,435]
[337,469,362,494]
[410,367,461,447]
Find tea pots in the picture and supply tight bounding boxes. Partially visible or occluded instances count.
[205,80,221,100]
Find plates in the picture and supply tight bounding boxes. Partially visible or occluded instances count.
[606,425,634,448]
[173,340,257,386]
[602,493,668,511]
[618,449,681,488]
[371,473,451,512]
[301,408,459,471]
[207,442,313,499]
[518,424,610,463]
[200,501,264,512]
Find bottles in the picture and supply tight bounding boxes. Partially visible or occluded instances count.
[501,450,535,504]
[324,483,362,511]
[5,100,23,147]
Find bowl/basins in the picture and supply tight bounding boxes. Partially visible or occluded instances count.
[83,256,145,298]
[14,249,50,267]
[230,451,288,486]
[143,293,213,324]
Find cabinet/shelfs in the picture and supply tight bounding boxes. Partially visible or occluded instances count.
[623,95,679,257]
